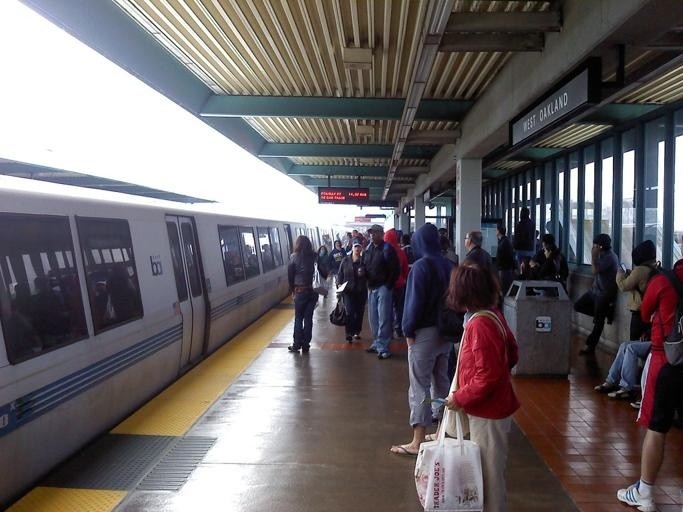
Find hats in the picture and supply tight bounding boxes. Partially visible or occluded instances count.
[367,224,383,232]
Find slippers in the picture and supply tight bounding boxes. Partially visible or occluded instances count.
[389,444,417,458]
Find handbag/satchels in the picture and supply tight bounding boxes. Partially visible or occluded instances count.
[437,301,463,342]
[312,263,329,295]
[330,296,351,326]
[415,368,484,512]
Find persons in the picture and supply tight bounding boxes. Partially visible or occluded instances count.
[571,233,620,356]
[287,224,408,360]
[492,208,569,296]
[615,234,682,509]
[386,220,517,510]
[0,238,283,365]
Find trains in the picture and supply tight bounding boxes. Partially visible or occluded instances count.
[0,174,343,511]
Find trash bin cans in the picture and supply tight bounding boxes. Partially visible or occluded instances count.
[503,280,573,379]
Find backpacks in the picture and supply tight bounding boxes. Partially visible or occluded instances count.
[652,271,683,366]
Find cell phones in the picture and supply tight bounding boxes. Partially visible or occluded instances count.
[620,263,626,272]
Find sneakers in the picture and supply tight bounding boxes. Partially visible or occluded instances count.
[366,342,392,359]
[617,480,657,512]
[593,382,642,410]
